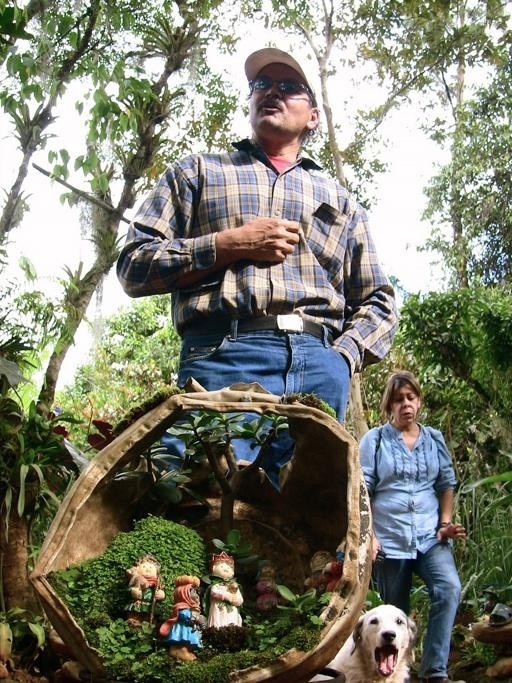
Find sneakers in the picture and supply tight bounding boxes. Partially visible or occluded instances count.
[429,677,466,683]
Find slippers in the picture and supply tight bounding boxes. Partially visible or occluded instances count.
[488,603,512,626]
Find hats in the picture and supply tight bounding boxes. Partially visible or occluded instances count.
[245,49,316,96]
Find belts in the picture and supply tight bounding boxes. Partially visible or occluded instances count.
[182,313,336,345]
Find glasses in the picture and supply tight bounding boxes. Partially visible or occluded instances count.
[249,76,315,107]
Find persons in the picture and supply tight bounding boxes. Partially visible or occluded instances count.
[123,537,346,662]
[354,370,468,683]
[115,48,398,495]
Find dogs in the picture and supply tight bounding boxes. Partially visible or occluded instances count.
[307,604,419,683]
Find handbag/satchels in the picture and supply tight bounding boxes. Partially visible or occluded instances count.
[368,551,386,593]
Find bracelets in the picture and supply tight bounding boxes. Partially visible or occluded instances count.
[441,522,452,527]
